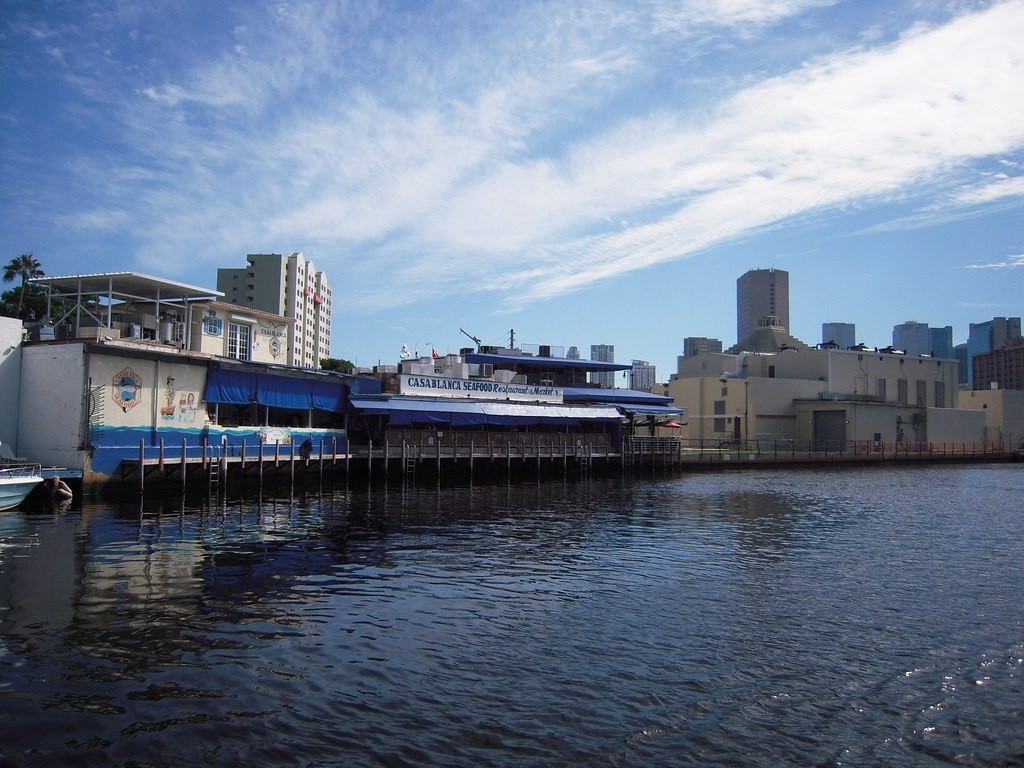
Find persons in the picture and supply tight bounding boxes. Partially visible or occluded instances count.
[900,429,904,441]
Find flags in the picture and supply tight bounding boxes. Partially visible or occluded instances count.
[416,351,421,359]
[433,349,439,357]
[400,342,411,358]
